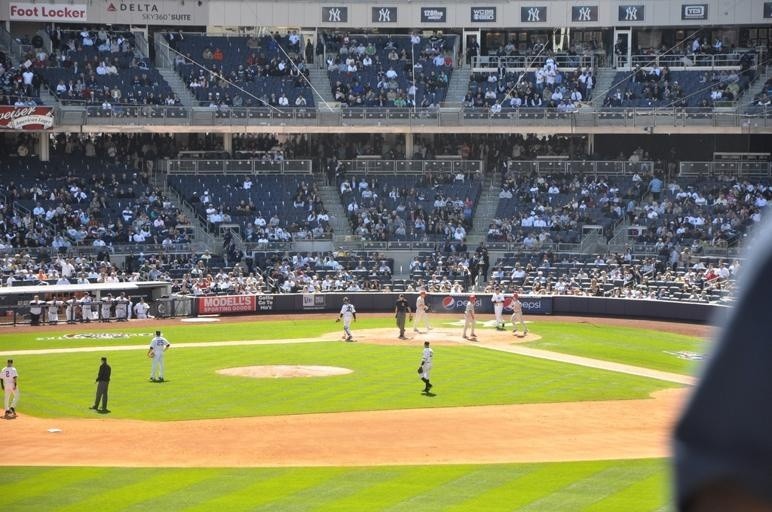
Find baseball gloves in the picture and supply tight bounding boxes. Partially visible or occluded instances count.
[409,315,412,321]
[148,353,155,357]
[417,368,423,374]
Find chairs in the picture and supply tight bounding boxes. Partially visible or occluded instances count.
[1,30,771,308]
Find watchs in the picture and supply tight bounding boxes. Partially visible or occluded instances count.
[491,287,505,328]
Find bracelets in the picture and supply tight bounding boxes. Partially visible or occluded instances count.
[338,318,341,320]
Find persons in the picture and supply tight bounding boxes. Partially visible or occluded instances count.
[394,294,413,340]
[418,342,434,393]
[334,297,357,342]
[1,21,771,325]
[89,356,112,413]
[414,291,432,332]
[1,358,20,417]
[462,295,476,338]
[147,331,170,383]
[504,293,528,335]
